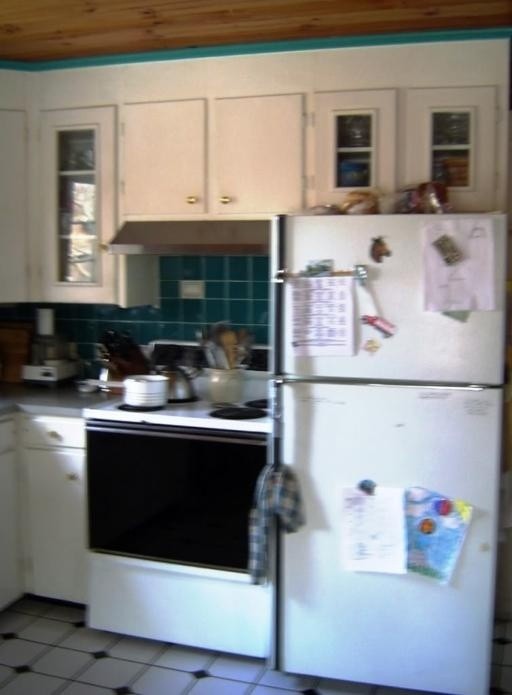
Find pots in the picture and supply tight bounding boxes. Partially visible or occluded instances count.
[124,375,170,408]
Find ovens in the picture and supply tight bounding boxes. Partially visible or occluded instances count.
[84,419,269,577]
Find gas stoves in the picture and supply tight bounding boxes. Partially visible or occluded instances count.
[83,391,279,434]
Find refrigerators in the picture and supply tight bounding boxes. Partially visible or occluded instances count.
[274,210,511,695]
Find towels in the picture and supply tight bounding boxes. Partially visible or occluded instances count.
[247,463,302,584]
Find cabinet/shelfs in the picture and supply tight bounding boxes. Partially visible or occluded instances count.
[122,95,304,218]
[0,448,29,609]
[401,88,495,210]
[23,423,89,606]
[38,106,112,303]
[316,91,397,211]
[0,110,28,301]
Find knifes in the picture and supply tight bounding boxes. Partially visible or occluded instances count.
[99,329,139,357]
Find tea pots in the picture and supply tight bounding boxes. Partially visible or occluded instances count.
[152,365,202,399]
